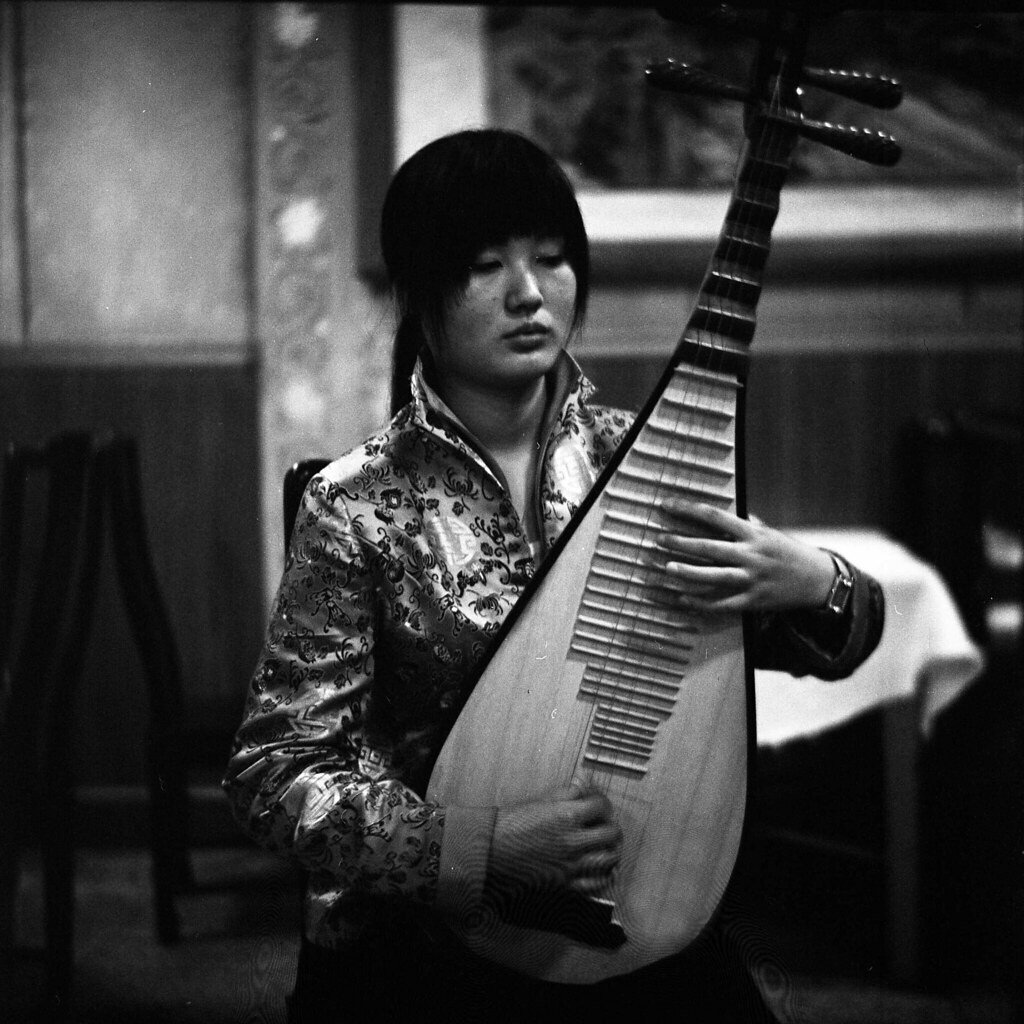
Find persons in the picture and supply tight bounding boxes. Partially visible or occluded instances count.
[219,126,887,1024]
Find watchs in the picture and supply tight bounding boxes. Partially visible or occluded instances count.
[810,555,852,617]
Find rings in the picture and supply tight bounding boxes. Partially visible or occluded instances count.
[572,778,588,797]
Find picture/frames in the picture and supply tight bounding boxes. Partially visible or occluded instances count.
[357,6,1021,288]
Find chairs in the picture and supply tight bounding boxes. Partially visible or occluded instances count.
[0,420,256,983]
[282,456,773,1024]
